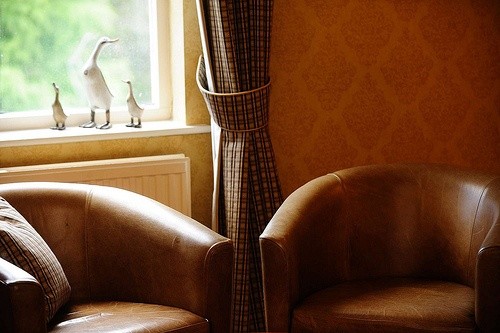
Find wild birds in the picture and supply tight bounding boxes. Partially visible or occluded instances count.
[51,82,68,130]
[122,80,145,129]
[78,36,119,130]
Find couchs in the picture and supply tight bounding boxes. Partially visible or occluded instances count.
[258,161,500,333]
[0,180,234,333]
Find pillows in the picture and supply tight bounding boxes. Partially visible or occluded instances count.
[0,196,73,323]
